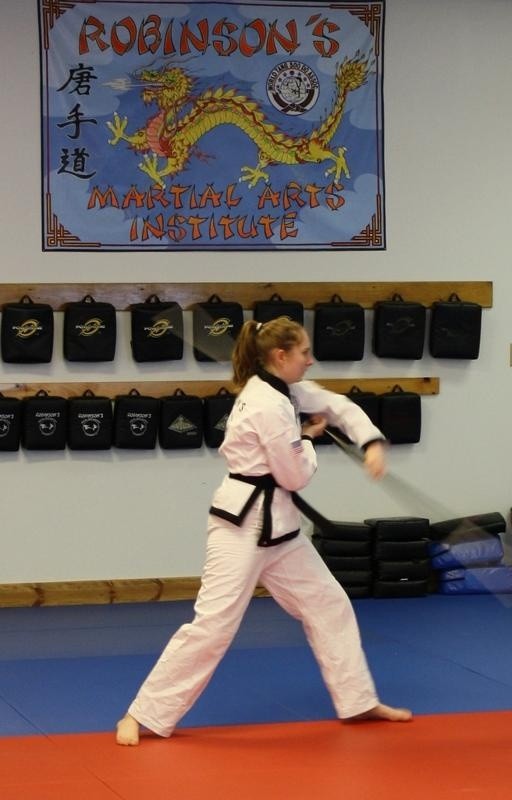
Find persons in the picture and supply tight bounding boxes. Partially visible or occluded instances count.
[116,317,415,746]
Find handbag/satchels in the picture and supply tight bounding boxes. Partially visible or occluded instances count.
[0,391,21,451]
[64,295,116,362]
[339,385,376,445]
[159,388,203,449]
[429,292,481,359]
[192,293,243,362]
[22,389,66,450]
[301,413,333,445]
[204,387,237,448]
[254,293,303,327]
[129,293,183,362]
[313,294,364,361]
[376,384,421,444]
[67,388,113,450]
[372,294,425,359]
[1,294,53,363]
[114,388,158,449]
